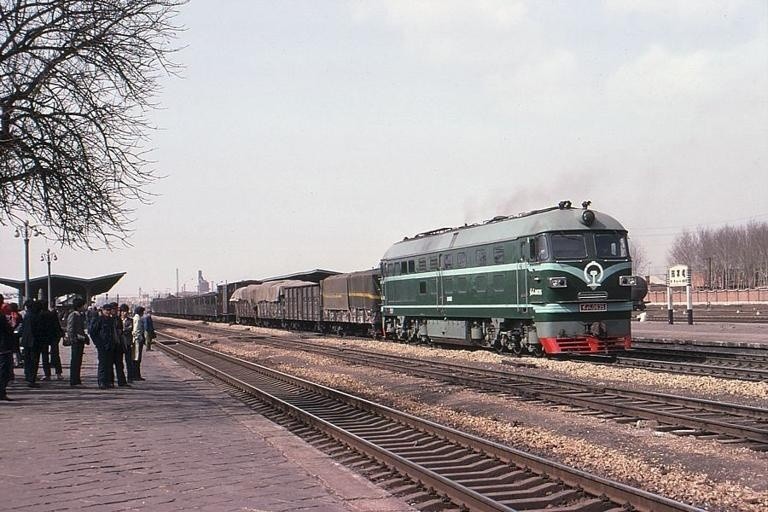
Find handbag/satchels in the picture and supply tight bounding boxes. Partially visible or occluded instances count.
[62,331,90,346]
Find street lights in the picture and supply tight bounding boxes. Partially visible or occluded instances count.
[14,219,38,304]
[40,247,57,311]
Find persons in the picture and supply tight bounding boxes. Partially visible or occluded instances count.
[0,294,155,400]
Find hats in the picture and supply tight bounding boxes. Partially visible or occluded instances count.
[110,302,119,309]
[103,304,112,310]
[120,304,129,313]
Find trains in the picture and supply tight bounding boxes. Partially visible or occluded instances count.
[149,199,636,362]
[629,274,650,311]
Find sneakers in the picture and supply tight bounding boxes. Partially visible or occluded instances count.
[28,382,40,387]
[40,375,51,381]
[99,377,146,389]
[56,373,64,379]
[72,382,87,388]
